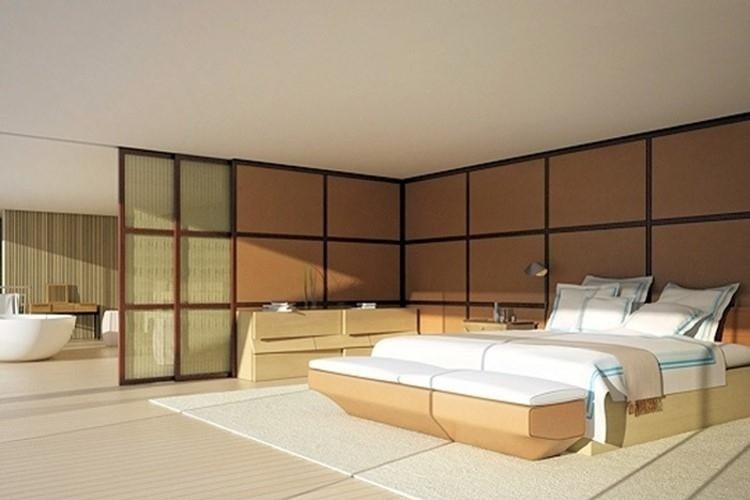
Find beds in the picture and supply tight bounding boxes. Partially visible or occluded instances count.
[378,305,750,456]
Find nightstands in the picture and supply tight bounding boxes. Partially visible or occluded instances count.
[463,318,534,334]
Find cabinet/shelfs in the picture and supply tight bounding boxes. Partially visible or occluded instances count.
[236,308,418,381]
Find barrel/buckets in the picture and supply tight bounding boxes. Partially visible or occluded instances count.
[496,307,513,322]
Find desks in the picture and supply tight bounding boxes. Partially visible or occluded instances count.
[28,303,98,341]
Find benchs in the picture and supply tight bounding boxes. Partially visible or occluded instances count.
[307,354,588,461]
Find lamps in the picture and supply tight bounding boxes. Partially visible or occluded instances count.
[523,261,549,277]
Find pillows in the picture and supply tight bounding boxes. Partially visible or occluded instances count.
[545,273,739,342]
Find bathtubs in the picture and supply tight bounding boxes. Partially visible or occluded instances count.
[0,314,76,361]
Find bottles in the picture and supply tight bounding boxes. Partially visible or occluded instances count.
[493,302,499,323]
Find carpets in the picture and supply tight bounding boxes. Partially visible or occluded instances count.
[146,381,750,500]
